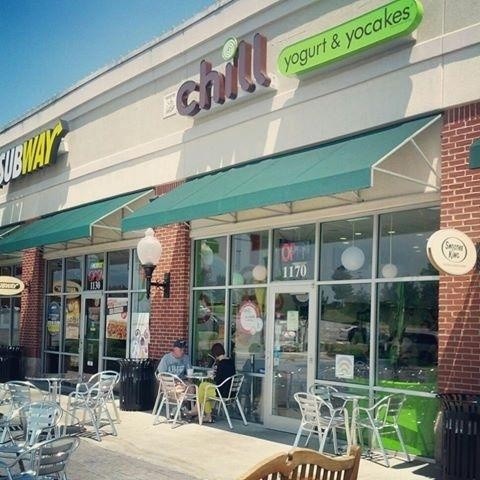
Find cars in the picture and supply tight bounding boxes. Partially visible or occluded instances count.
[340,326,438,364]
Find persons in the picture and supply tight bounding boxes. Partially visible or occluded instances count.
[184,342,238,423]
[238,342,264,407]
[155,337,195,416]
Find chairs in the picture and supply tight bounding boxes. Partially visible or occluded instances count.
[0,369,125,480]
[151,367,414,469]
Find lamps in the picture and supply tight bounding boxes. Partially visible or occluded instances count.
[135,226,171,300]
[339,213,399,279]
[252,230,267,283]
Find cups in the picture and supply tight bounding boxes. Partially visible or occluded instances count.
[187,369,193,376]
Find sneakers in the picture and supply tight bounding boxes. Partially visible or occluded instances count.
[183,410,198,417]
[202,417,212,423]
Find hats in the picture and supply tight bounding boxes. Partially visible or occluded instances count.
[174,339,188,349]
